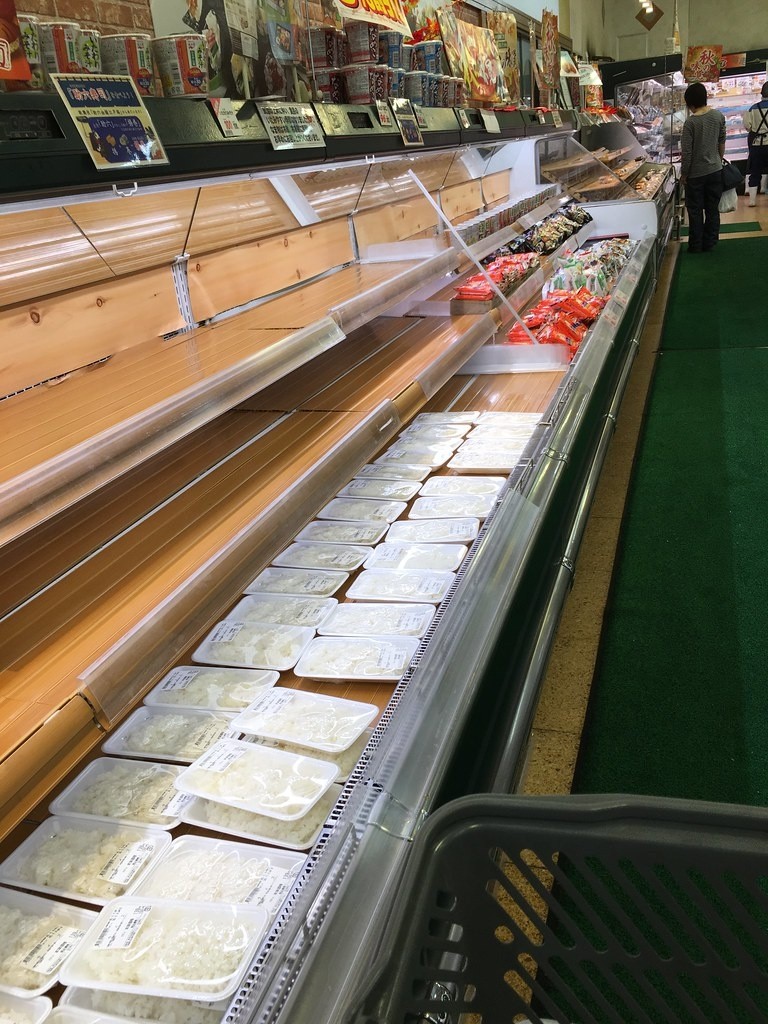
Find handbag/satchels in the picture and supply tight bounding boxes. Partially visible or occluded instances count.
[720,158,745,192]
[717,186,738,213]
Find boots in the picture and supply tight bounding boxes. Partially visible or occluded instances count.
[748,186,758,207]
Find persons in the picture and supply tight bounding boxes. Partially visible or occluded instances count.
[745,81,768,207]
[680,82,726,254]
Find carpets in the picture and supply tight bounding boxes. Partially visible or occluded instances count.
[670,221,763,238]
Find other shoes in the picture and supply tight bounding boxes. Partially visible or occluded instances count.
[702,246,716,252]
[687,247,702,252]
